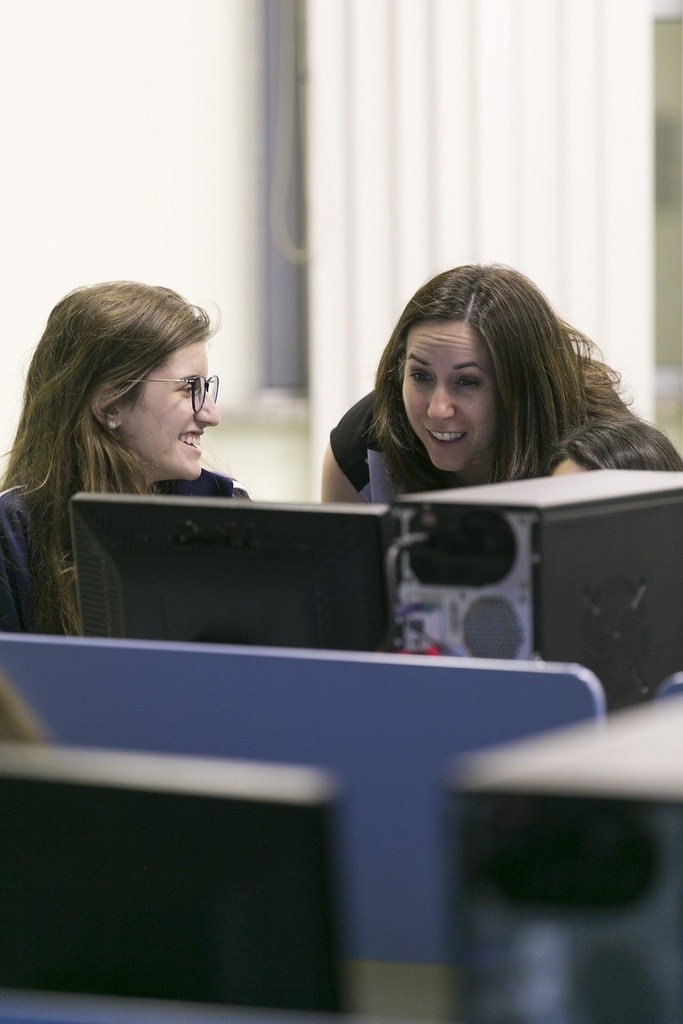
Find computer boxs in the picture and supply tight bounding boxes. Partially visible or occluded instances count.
[385,469,683,1018]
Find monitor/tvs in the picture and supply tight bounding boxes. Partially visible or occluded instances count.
[0,489,390,1006]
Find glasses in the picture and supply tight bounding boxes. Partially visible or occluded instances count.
[122,375,220,413]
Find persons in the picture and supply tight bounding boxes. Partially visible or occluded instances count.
[321,263,646,529]
[0,283,269,643]
